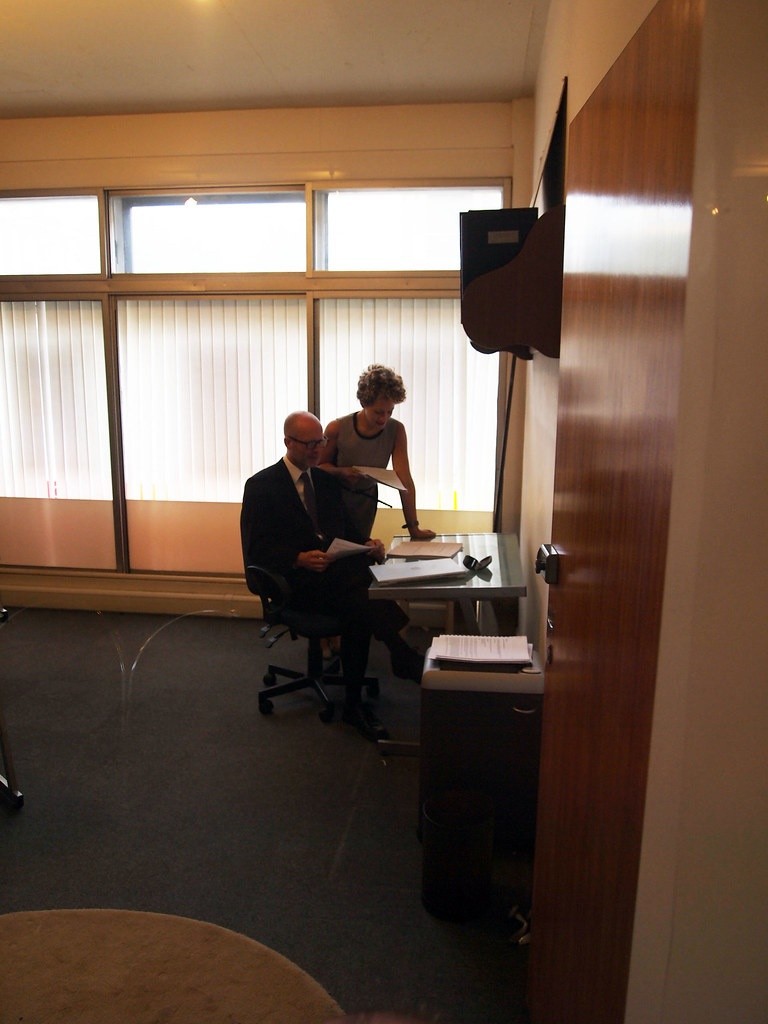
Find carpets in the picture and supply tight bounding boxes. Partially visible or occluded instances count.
[0,910,347,1024]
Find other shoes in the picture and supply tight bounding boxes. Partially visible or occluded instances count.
[322,646,333,661]
[333,643,341,656]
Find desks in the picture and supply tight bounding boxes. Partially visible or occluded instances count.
[369,533,526,755]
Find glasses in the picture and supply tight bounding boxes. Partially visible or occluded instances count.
[286,436,329,449]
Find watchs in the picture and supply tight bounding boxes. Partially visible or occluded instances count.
[402,521,419,529]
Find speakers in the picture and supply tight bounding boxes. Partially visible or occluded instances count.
[460,206,538,300]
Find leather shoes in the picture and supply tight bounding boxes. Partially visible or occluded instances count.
[390,646,424,686]
[341,700,391,744]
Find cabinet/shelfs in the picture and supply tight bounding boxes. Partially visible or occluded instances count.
[418,646,545,843]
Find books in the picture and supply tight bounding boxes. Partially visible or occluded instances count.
[352,465,408,491]
[319,538,373,561]
[428,634,533,667]
[369,542,466,584]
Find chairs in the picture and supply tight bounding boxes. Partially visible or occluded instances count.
[244,566,377,723]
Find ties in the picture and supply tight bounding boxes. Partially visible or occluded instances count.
[300,472,330,544]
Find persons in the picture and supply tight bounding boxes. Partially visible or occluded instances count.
[239,364,437,737]
